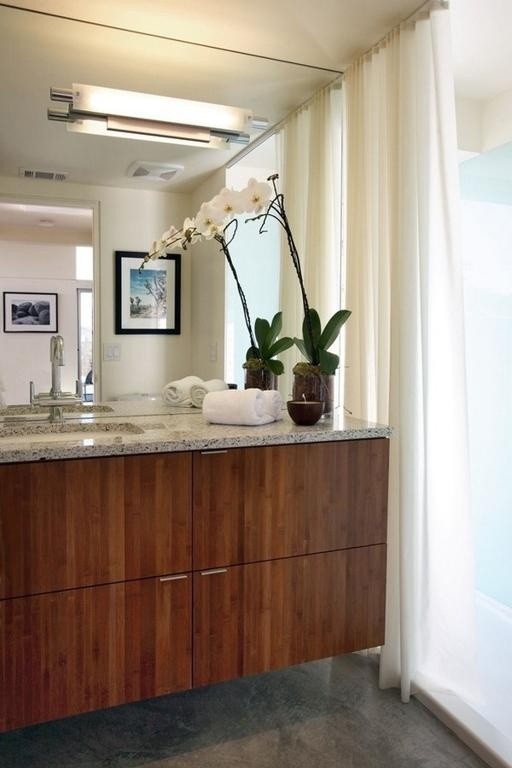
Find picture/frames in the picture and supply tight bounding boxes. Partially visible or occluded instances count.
[113,249,182,335]
[2,291,59,334]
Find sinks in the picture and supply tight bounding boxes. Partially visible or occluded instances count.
[0,422,146,440]
[0,404,115,418]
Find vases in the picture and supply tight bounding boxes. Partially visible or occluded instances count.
[292,363,334,414]
[245,367,278,389]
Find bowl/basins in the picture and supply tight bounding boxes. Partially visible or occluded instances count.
[287,400,326,426]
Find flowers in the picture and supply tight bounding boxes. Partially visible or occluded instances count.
[181,172,352,374]
[138,217,295,376]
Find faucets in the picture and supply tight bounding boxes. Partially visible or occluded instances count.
[48,334,62,397]
[47,405,66,424]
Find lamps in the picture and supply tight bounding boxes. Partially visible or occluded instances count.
[45,104,270,149]
[50,81,270,137]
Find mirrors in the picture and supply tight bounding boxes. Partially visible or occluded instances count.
[0,3,346,421]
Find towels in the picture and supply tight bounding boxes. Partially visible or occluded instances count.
[160,375,229,409]
[202,388,282,426]
